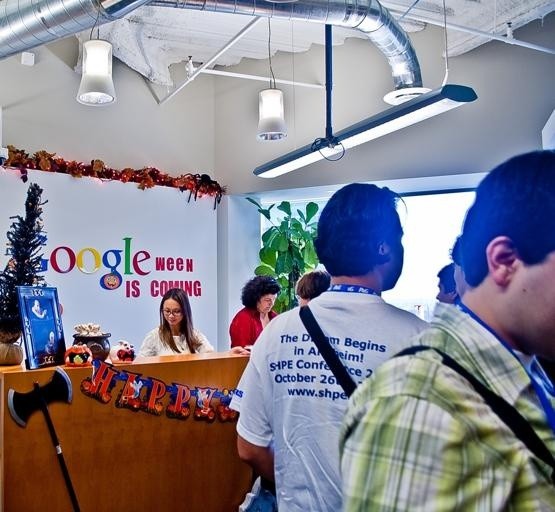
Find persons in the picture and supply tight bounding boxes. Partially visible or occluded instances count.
[294,270,332,305]
[227,181,432,512]
[229,274,281,352]
[434,261,458,305]
[450,234,465,297]
[335,147,554,509]
[138,288,250,356]
[44,331,57,356]
[30,300,47,319]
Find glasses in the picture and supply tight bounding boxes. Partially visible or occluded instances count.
[162,310,184,316]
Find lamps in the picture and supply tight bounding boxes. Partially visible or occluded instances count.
[257,16,287,141]
[253,1,477,180]
[76,1,117,105]
